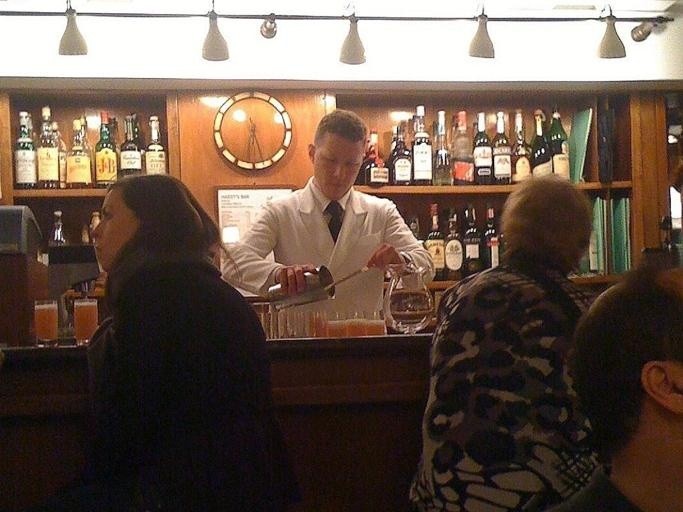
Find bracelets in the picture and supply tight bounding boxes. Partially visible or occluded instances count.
[400,251,411,264]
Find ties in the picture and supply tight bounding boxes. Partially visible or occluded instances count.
[326,201,344,243]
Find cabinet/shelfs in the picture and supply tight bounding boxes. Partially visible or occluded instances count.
[0,88,182,299]
[325,88,645,331]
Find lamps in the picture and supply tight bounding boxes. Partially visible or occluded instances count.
[59,1,653,65]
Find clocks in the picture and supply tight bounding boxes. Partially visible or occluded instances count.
[213,90,292,170]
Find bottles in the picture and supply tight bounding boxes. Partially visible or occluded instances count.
[91,212,100,230]
[47,211,65,247]
[411,203,506,281]
[354,103,571,185]
[14,106,168,188]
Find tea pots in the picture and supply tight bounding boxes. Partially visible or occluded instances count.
[384,264,434,334]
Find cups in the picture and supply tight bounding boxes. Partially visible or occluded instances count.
[256,309,384,340]
[34,300,58,348]
[268,265,336,313]
[74,299,98,346]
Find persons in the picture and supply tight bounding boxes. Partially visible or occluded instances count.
[73,173,302,512]
[221,109,437,338]
[555,267,682,512]
[407,174,610,512]
